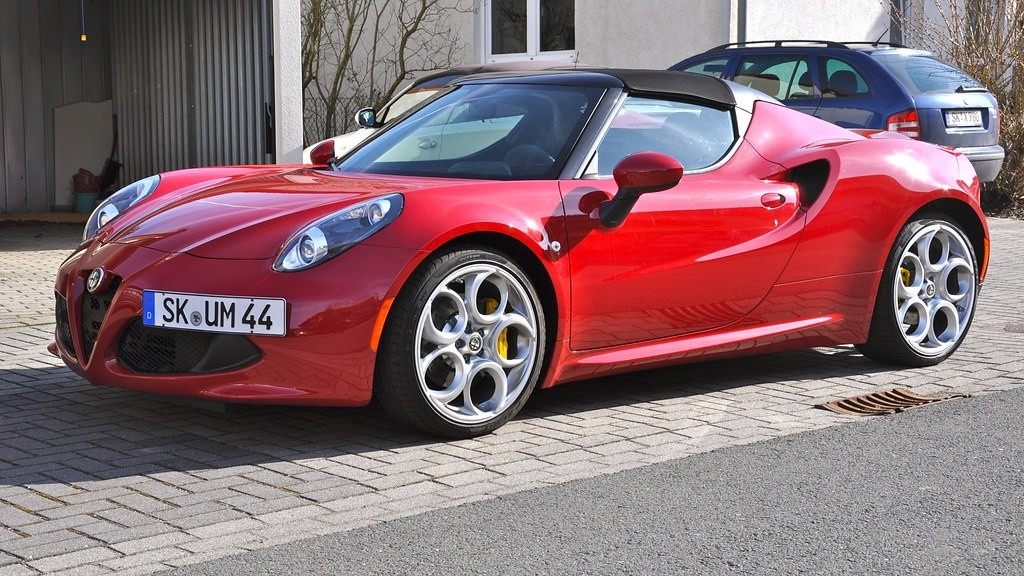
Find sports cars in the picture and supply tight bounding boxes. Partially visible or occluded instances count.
[48,66,993,439]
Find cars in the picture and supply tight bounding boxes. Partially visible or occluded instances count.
[303,58,700,166]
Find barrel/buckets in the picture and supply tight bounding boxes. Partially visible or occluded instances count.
[73,192,102,213]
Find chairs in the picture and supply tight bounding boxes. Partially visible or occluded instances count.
[829,71,858,96]
[750,74,780,98]
[790,72,815,98]
[660,112,717,168]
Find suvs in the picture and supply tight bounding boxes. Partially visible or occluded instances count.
[659,37,1006,181]
